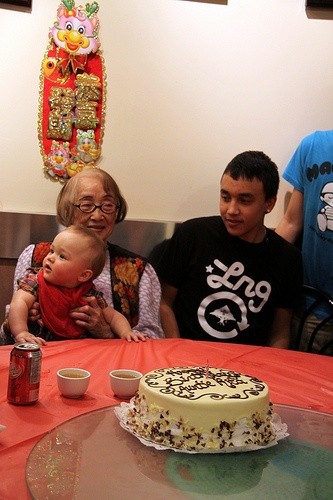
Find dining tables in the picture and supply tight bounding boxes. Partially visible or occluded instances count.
[0,337,333,500]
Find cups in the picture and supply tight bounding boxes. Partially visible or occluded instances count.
[109,369,143,399]
[57,368,91,399]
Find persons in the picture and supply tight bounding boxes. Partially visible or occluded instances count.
[154,151,306,351]
[276,129,333,355]
[0,168,164,348]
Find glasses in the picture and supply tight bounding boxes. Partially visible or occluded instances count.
[71,200,120,214]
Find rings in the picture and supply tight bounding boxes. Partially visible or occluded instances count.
[88,316,93,321]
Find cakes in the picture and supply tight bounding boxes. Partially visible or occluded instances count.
[125,366,277,451]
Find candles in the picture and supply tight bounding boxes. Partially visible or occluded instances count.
[205,360,209,379]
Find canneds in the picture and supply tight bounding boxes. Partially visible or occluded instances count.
[6,343,42,405]
